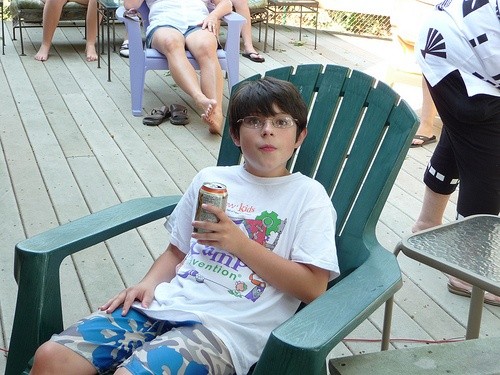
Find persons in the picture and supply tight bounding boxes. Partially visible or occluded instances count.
[410,0,500,307]
[120,0,140,57]
[231,0,266,62]
[33,0,105,62]
[123,0,232,135]
[408,67,440,150]
[28,77,341,375]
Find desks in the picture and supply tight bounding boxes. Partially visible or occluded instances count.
[381,214,499,354]
[259,0,320,50]
[96,0,142,82]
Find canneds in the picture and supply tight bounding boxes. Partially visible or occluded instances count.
[193,182,228,233]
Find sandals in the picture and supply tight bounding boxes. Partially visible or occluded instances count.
[168,103,190,124]
[120,40,130,57]
[143,105,172,126]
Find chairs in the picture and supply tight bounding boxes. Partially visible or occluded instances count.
[7,63,422,374]
[8,0,107,56]
[115,3,248,117]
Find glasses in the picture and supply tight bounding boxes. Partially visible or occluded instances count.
[237,116,298,128]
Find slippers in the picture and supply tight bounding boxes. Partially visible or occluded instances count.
[242,51,265,63]
[448,281,500,306]
[410,135,437,148]
